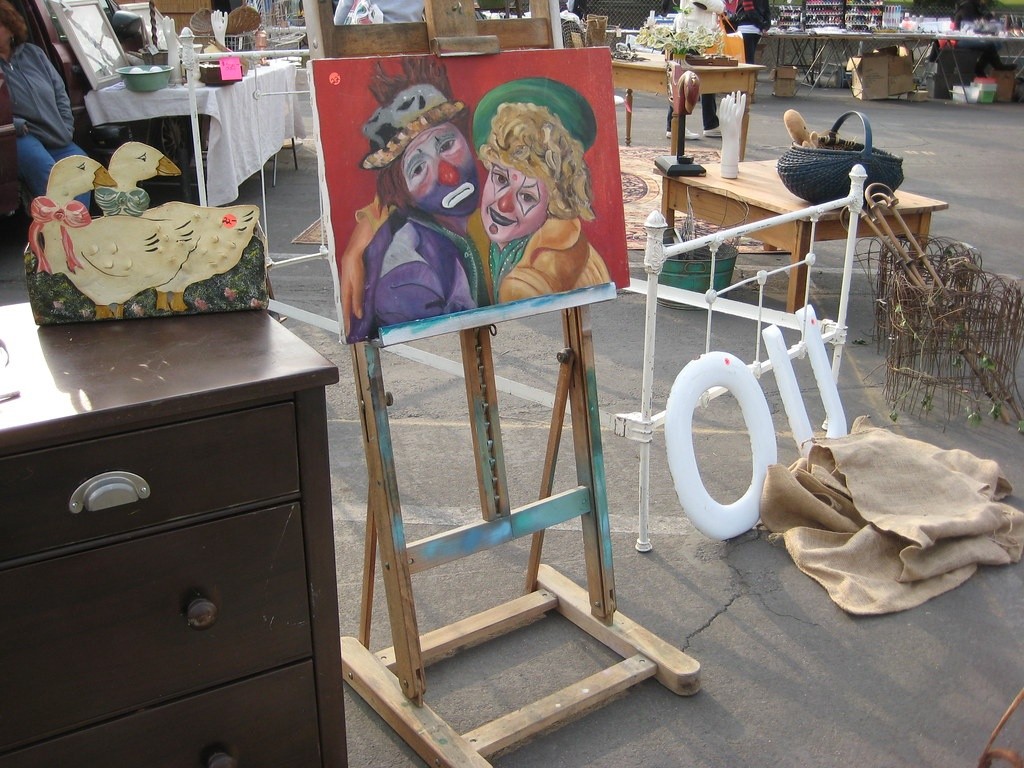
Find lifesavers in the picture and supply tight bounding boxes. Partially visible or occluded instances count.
[761,303,848,459]
[664,350,778,543]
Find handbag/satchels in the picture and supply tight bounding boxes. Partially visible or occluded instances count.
[704,14,745,63]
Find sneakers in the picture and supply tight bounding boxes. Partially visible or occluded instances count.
[665,126,700,140]
[703,126,723,137]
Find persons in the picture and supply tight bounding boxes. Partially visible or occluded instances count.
[723,0,771,64]
[667,0,726,139]
[333,0,424,25]
[954,1,1017,78]
[559,0,584,31]
[0,0,91,213]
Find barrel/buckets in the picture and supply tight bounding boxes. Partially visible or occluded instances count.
[657,227,739,312]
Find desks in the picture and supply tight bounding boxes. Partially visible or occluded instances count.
[654,158,949,314]
[85,59,300,208]
[611,51,767,159]
[762,33,1024,103]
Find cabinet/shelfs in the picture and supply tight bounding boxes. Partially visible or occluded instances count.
[0,302,348,768]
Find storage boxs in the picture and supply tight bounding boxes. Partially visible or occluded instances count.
[990,68,1014,101]
[906,90,930,102]
[846,54,889,101]
[970,82,997,103]
[952,86,980,102]
[771,65,800,98]
[873,46,912,95]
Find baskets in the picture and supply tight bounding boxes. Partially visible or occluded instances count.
[777,111,903,211]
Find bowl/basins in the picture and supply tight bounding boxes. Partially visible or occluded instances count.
[115,65,174,92]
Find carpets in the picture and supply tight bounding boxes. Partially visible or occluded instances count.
[289,146,789,254]
